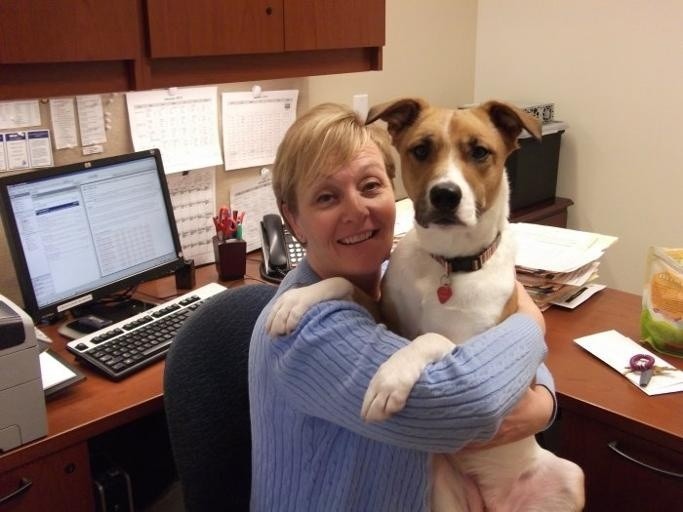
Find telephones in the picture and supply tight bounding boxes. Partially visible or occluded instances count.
[261,214,309,280]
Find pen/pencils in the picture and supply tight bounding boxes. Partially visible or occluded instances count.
[213,207,246,241]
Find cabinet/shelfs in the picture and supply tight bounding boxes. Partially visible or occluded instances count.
[147,1,387,92]
[0,0,145,100]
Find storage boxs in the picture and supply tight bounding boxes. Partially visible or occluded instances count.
[502,121,571,211]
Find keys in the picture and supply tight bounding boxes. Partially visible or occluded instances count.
[650,365,678,371]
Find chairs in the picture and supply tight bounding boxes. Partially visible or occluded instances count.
[163,278,280,511]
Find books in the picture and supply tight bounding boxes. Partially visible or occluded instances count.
[387,197,620,314]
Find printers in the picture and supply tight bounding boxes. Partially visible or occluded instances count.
[0,293,48,455]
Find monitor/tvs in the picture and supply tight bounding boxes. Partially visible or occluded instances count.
[0,148,185,322]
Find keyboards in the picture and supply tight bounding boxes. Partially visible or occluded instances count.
[65,282,228,381]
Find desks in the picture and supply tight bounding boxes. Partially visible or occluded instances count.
[0,248,683,510]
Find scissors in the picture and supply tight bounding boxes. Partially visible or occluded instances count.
[219,208,237,236]
